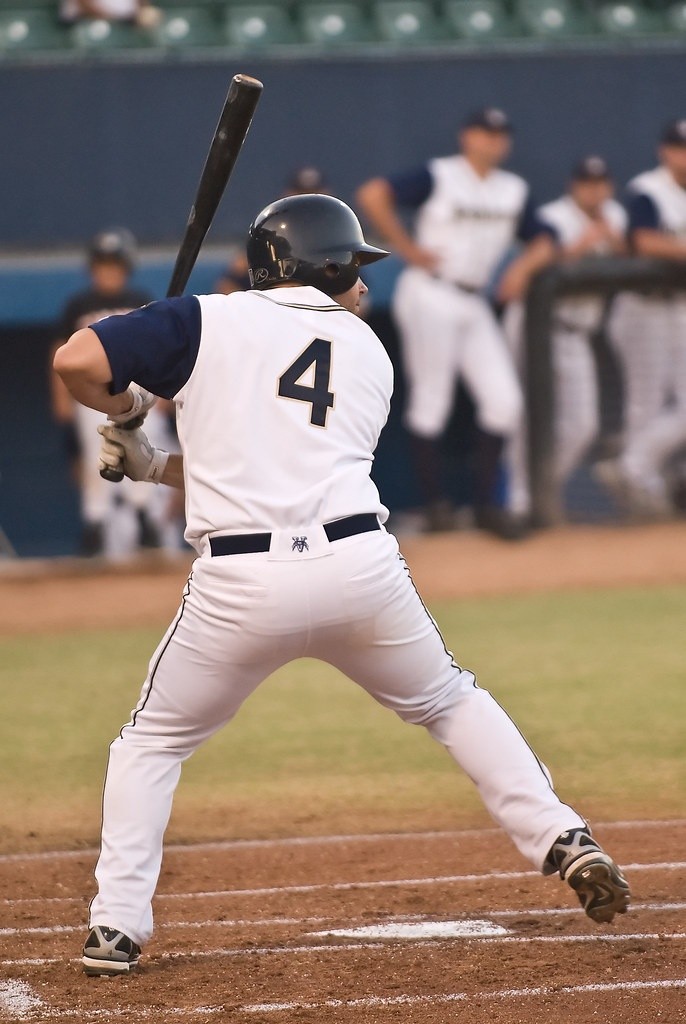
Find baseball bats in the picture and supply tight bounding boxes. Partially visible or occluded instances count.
[100,73,266,484]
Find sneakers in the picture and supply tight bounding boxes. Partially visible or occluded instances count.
[81,924,143,971]
[552,827,630,926]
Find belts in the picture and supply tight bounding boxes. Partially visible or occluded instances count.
[207,512,382,556]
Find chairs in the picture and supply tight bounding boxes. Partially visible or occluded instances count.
[0,0,686,42]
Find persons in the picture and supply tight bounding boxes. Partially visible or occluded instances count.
[498,150,627,524]
[53,234,185,564]
[53,192,633,972]
[598,118,686,523]
[221,109,532,537]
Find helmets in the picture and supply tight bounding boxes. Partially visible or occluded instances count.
[247,192,391,294]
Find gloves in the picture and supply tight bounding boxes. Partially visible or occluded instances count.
[94,423,171,485]
[107,383,158,423]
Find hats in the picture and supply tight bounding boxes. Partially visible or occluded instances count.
[662,118,686,147]
[469,108,516,137]
[573,156,612,182]
